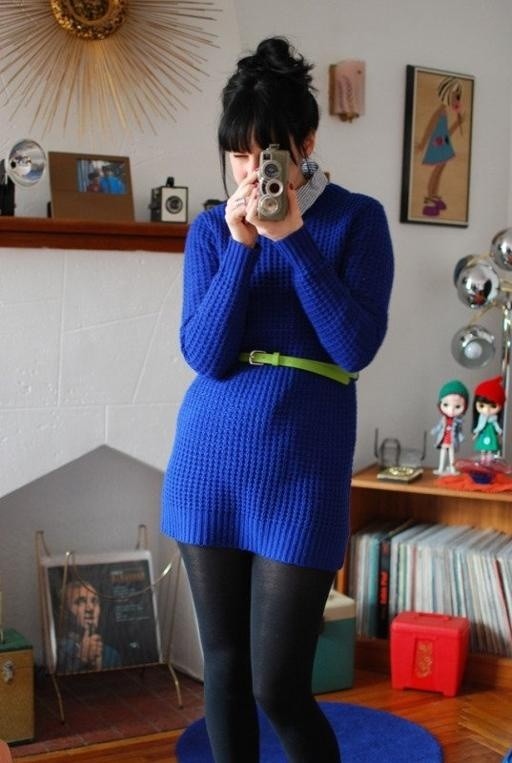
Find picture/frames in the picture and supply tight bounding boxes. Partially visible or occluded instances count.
[48,150,136,222]
[399,65,475,228]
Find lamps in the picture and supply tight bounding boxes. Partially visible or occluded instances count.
[452,227,512,466]
[0,139,46,216]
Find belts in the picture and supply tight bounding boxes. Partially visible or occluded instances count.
[240,350,359,385]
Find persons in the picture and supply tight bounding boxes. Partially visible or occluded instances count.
[55,580,118,672]
[155,36,392,760]
[472,376,505,464]
[85,164,124,195]
[428,380,468,475]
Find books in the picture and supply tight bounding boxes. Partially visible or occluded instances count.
[348,516,512,659]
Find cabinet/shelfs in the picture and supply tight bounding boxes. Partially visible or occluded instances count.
[336,463,512,690]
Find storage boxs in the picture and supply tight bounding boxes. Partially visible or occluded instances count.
[0,649,35,745]
[311,584,357,695]
[390,611,472,697]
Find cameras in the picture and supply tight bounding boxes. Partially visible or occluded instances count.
[254,143,289,223]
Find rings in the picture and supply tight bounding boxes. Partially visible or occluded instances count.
[235,198,245,207]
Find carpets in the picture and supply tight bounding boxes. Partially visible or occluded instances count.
[176,700,442,763]
[8,663,206,759]
